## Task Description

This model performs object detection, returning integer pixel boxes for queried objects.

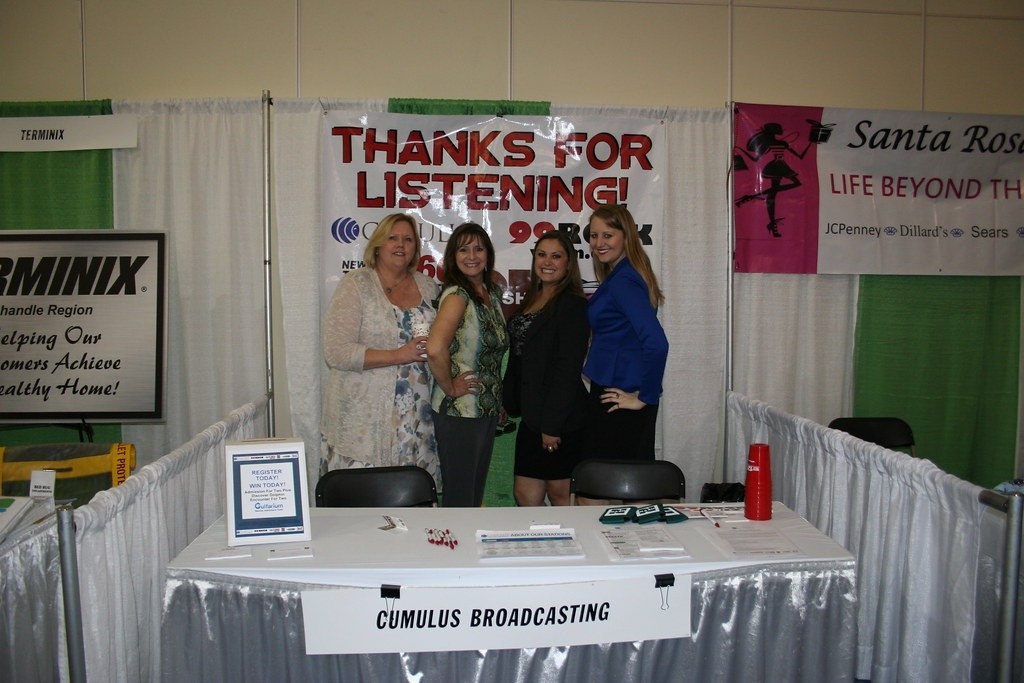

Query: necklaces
[377,272,408,293]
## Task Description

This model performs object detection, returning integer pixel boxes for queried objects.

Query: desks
[162,501,855,682]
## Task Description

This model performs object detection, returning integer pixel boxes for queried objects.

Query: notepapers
[637,540,684,552]
[529,520,561,530]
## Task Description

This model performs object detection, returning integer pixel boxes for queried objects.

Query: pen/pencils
[424,527,459,549]
[699,508,722,530]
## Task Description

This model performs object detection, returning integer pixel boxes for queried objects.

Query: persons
[424,224,510,508]
[319,212,444,508]
[575,204,669,506]
[502,231,591,506]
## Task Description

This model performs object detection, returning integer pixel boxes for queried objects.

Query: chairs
[829,417,916,459]
[315,465,439,507]
[569,459,686,501]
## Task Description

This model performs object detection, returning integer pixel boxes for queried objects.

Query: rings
[416,345,421,350]
[616,393,619,398]
[470,384,471,388]
[616,404,620,409]
[548,446,552,450]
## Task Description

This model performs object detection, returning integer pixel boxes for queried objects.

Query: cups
[745,443,773,521]
